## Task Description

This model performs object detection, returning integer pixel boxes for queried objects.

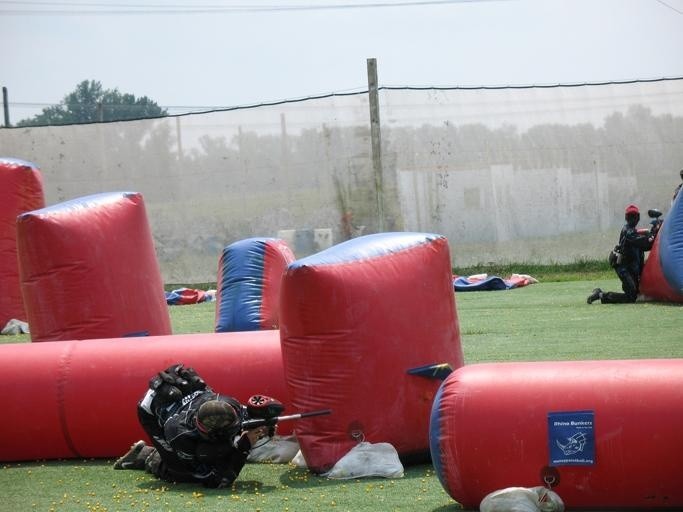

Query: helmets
[196,400,242,438]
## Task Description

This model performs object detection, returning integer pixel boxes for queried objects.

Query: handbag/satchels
[608,245,625,268]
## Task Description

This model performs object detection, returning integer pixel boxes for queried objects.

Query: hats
[625,204,639,215]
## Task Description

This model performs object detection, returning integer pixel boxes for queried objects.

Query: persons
[114,392,277,489]
[587,204,658,305]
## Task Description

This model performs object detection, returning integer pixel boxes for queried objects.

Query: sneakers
[114,440,145,469]
[587,288,602,304]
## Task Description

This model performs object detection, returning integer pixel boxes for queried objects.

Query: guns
[240,395,333,440]
[649,209,663,235]
